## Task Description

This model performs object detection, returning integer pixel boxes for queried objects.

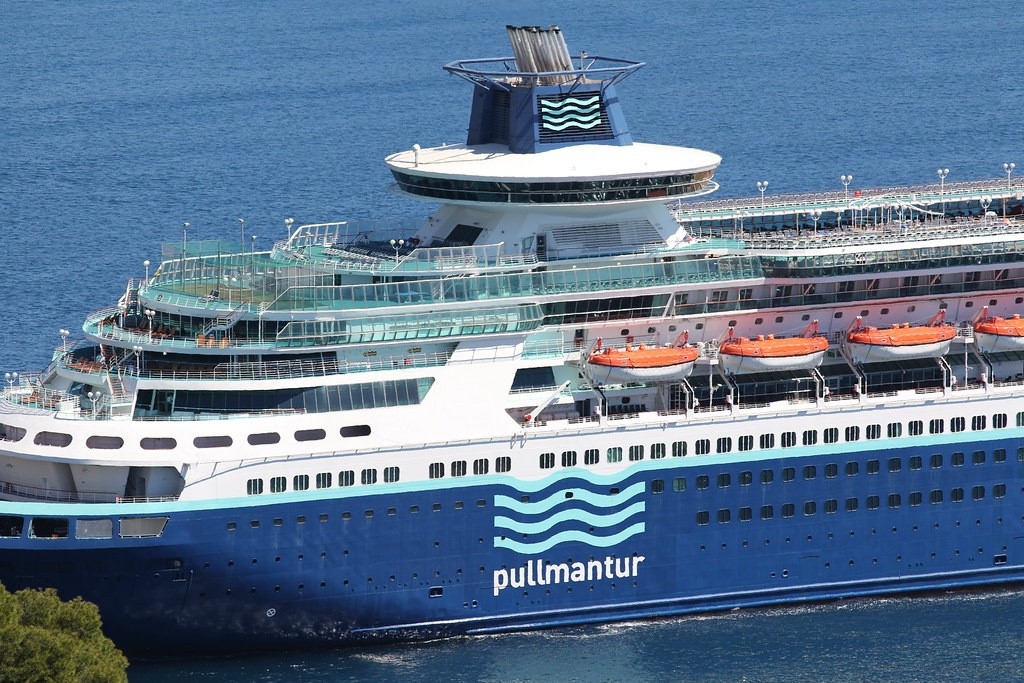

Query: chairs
[319,237,538,269]
[533,177,1024,301]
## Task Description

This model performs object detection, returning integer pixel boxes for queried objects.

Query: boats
[580,330,699,388]
[844,306,954,363]
[717,319,828,376]
[973,302,1024,351]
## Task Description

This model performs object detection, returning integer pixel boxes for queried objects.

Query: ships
[0,22,1022,637]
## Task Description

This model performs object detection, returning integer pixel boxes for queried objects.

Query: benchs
[65,356,102,374]
[127,318,176,342]
[18,391,63,409]
